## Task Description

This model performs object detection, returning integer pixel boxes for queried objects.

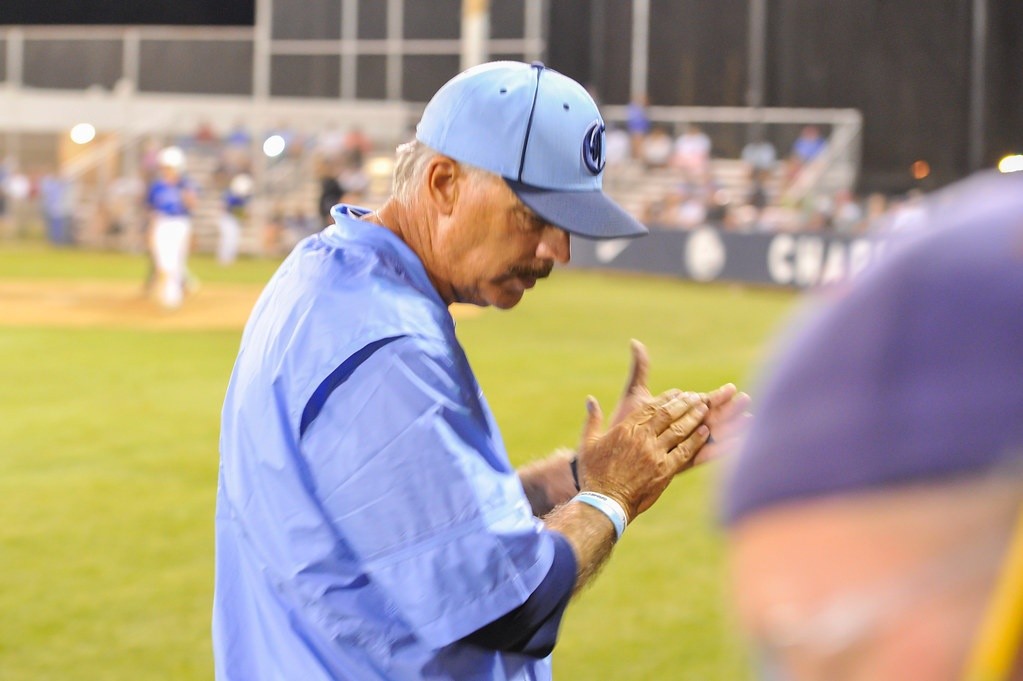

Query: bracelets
[571,457,581,492]
[571,488,628,541]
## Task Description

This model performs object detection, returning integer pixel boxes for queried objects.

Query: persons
[216,127,254,266]
[147,145,197,309]
[318,121,822,235]
[717,164,1022,681]
[40,165,79,242]
[213,58,755,681]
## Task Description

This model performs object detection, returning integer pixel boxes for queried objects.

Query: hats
[415,61,648,238]
